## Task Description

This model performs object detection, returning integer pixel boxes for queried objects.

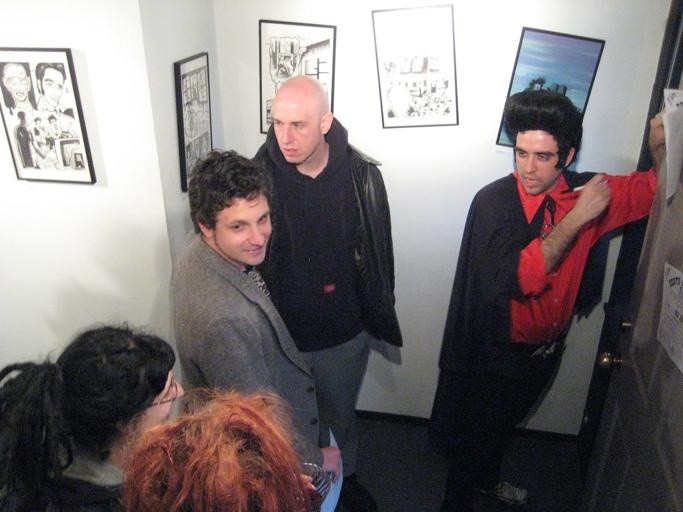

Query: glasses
[145,375,178,408]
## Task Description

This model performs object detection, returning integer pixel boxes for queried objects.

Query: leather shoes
[338,475,378,511]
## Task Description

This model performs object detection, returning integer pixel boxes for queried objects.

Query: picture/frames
[257,20,336,134]
[172,52,213,192]
[372,7,459,129]
[497,27,606,150]
[0,47,96,185]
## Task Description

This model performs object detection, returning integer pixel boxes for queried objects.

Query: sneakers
[492,481,528,507]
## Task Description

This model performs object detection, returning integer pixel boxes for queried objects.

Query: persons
[124,388,315,512]
[173,151,341,512]
[428,90,663,506]
[3,326,185,511]
[249,76,404,511]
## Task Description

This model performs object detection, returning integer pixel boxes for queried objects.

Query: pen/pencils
[573,179,608,193]
